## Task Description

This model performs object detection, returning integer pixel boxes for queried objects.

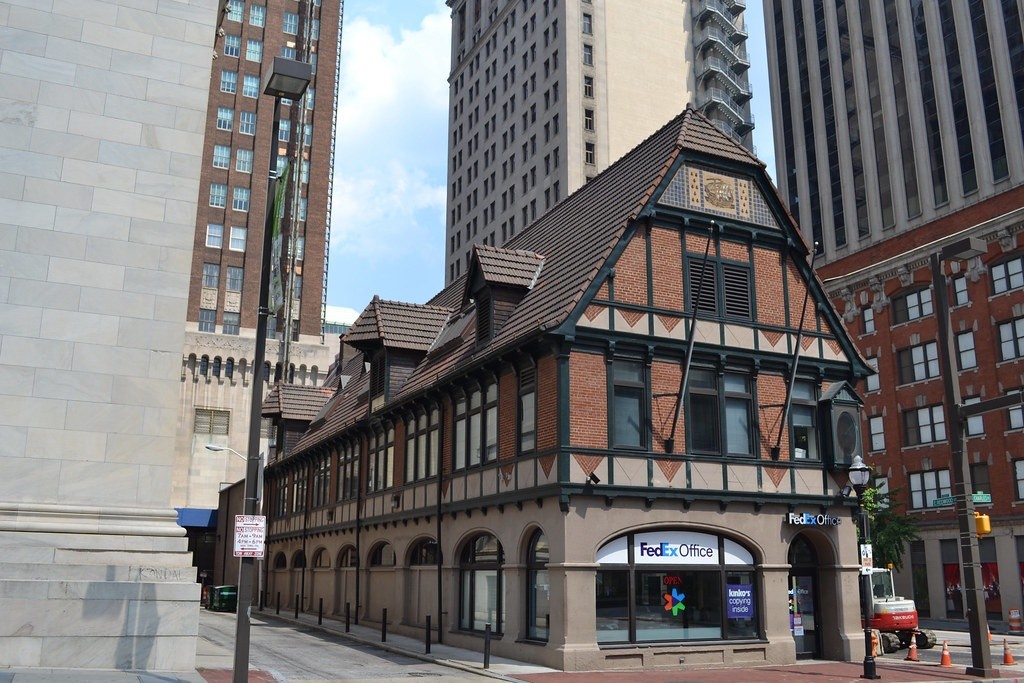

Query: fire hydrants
[871,631,878,658]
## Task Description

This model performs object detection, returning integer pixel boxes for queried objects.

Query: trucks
[206,585,237,613]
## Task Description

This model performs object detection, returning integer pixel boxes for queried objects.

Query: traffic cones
[936,640,953,668]
[987,623,994,643]
[903,634,921,662]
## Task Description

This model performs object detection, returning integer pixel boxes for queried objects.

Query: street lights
[205,56,312,683]
[928,248,1000,679]
[847,454,881,681]
[999,639,1017,666]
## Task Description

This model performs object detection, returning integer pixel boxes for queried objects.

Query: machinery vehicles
[858,568,938,655]
